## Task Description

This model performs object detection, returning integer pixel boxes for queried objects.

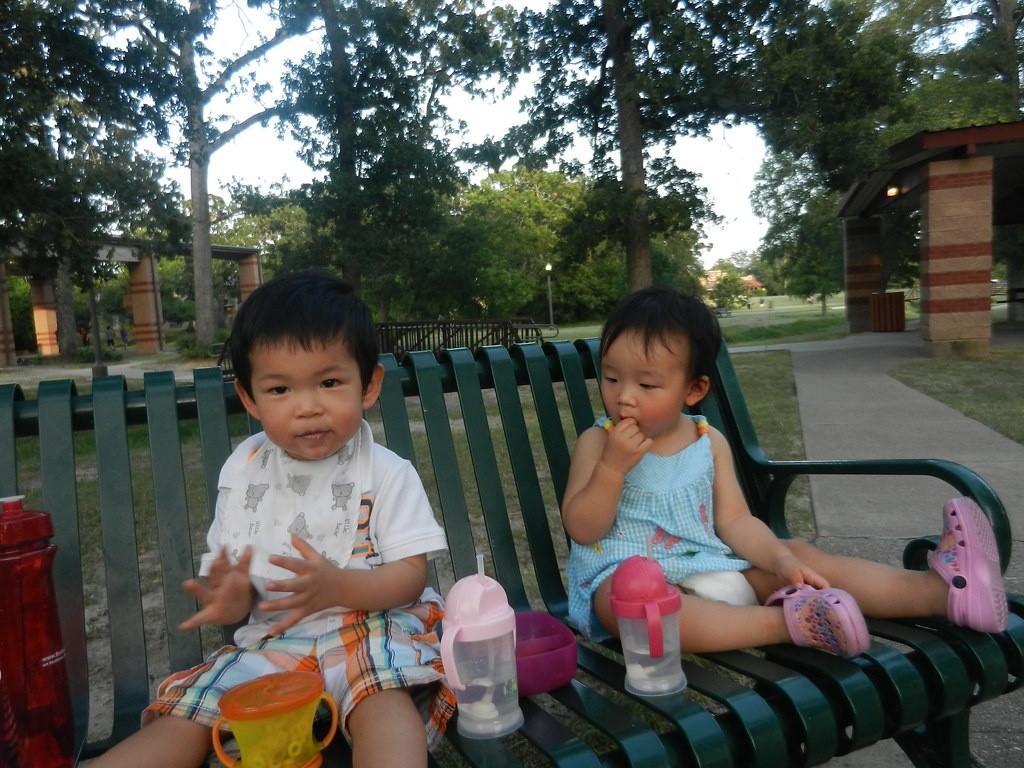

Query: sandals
[762,583,870,657]
[925,497,1009,635]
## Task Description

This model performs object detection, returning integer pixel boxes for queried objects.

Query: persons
[806,293,814,303]
[710,304,722,318]
[65,255,453,768]
[550,286,1014,662]
[759,296,765,307]
[79,326,91,348]
[104,323,116,350]
[120,323,128,350]
[746,295,752,310]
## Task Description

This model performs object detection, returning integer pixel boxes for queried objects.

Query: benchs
[0,337,1024,768]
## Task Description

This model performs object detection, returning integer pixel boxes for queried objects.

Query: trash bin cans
[871,290,906,333]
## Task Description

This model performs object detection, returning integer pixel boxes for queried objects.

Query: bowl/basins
[514,608,578,697]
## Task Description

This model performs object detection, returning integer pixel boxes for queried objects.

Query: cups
[610,536,694,695]
[436,554,524,740]
[212,670,338,768]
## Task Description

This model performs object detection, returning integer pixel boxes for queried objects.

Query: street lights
[545,262,556,330]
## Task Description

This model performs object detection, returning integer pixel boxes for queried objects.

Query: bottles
[0,491,79,767]
